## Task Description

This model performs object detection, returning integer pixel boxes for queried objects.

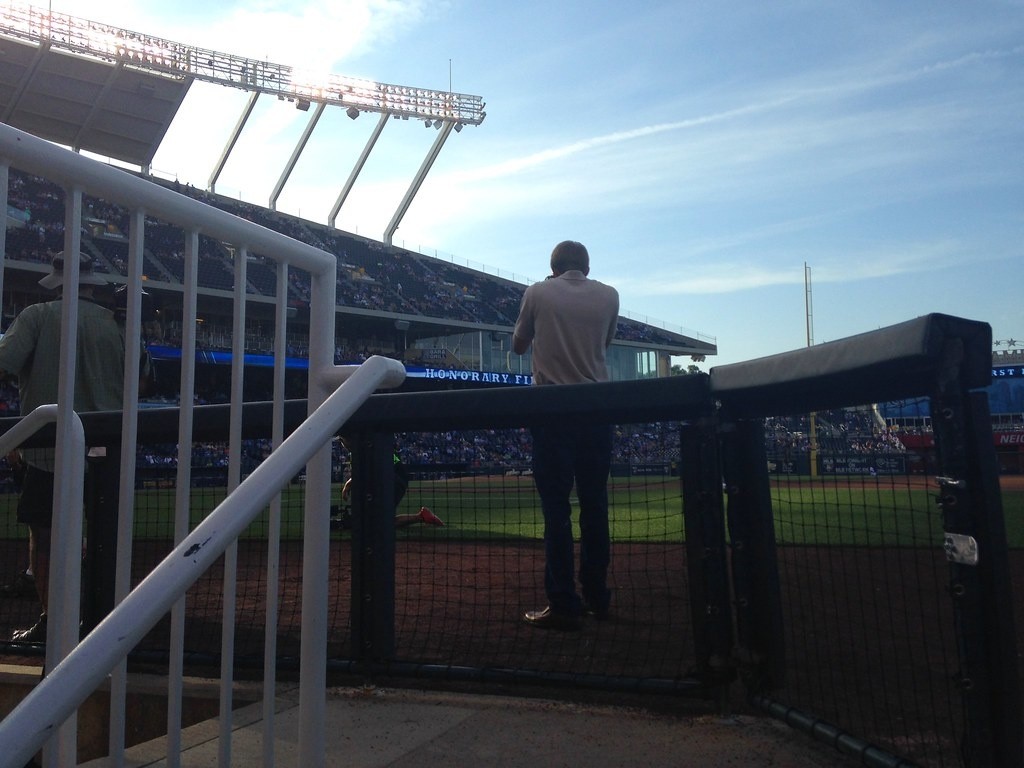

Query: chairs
[4,164,697,349]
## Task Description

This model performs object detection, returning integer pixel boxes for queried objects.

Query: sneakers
[420,507,445,526]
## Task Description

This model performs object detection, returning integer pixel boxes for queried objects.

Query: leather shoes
[523,604,579,631]
[583,607,609,620]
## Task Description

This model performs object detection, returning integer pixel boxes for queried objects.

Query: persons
[339,423,444,528]
[2,157,690,371]
[514,241,620,624]
[0,250,150,653]
[0,372,907,482]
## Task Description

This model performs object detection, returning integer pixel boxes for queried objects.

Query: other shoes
[11,620,50,645]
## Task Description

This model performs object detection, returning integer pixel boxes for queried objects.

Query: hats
[38,249,112,294]
